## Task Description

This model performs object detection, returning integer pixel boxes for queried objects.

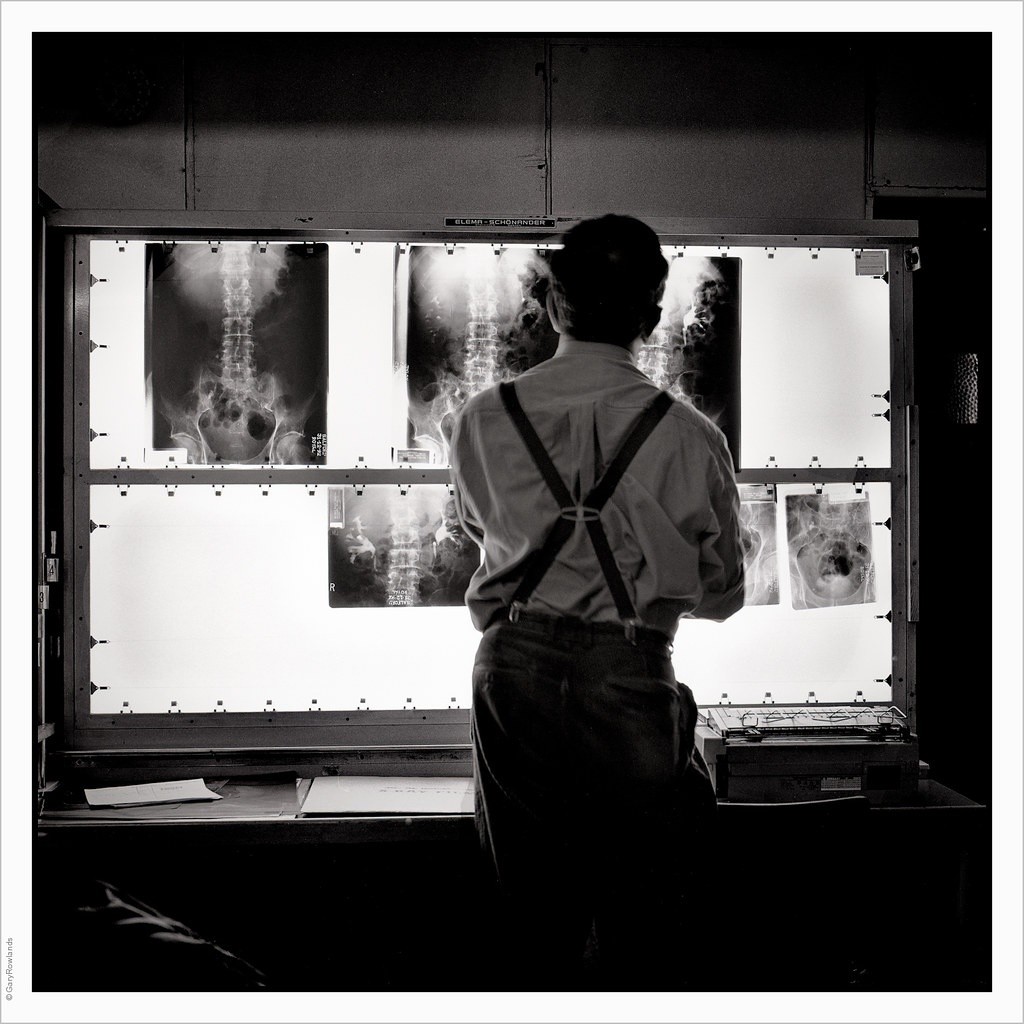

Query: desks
[37,771,998,841]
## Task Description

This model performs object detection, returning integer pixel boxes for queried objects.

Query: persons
[447,214,746,991]
